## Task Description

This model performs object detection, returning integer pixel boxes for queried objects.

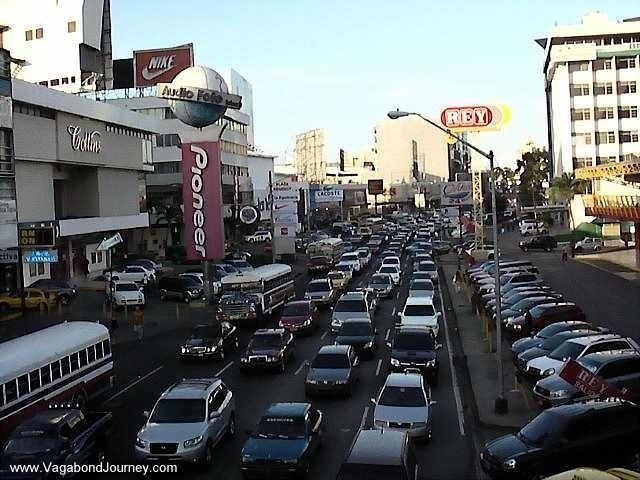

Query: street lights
[384,107,509,415]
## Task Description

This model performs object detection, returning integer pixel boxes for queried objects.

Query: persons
[524,306,532,336]
[561,244,568,264]
[453,270,462,294]
[132,305,145,341]
